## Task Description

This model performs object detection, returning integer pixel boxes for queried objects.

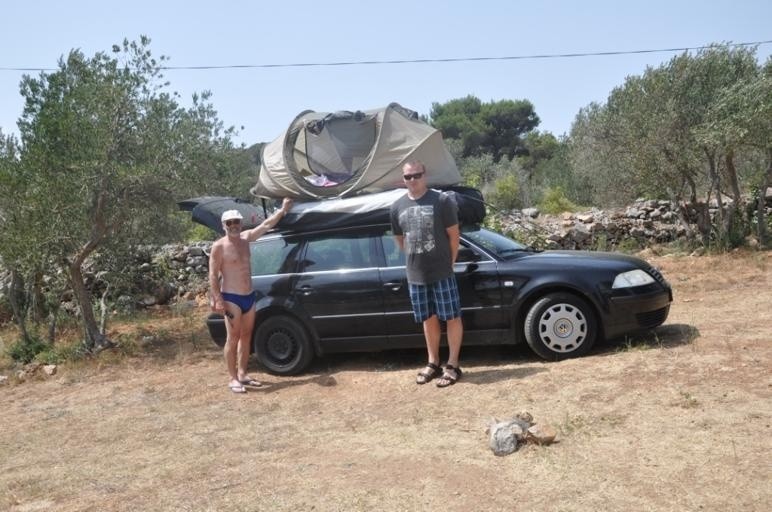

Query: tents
[248,101,466,200]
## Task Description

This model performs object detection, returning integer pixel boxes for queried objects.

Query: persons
[208,197,293,394]
[389,159,464,388]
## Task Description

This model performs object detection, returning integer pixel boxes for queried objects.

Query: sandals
[416,362,443,384]
[436,365,461,387]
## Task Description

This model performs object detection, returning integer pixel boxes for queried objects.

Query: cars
[175,194,673,376]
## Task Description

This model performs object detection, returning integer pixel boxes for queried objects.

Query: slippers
[239,378,262,386]
[229,382,247,393]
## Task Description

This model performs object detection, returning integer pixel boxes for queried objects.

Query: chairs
[381,238,403,266]
[304,249,346,271]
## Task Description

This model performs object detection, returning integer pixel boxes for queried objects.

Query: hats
[221,210,243,222]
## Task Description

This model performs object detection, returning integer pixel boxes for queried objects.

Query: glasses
[226,219,240,226]
[404,172,423,180]
